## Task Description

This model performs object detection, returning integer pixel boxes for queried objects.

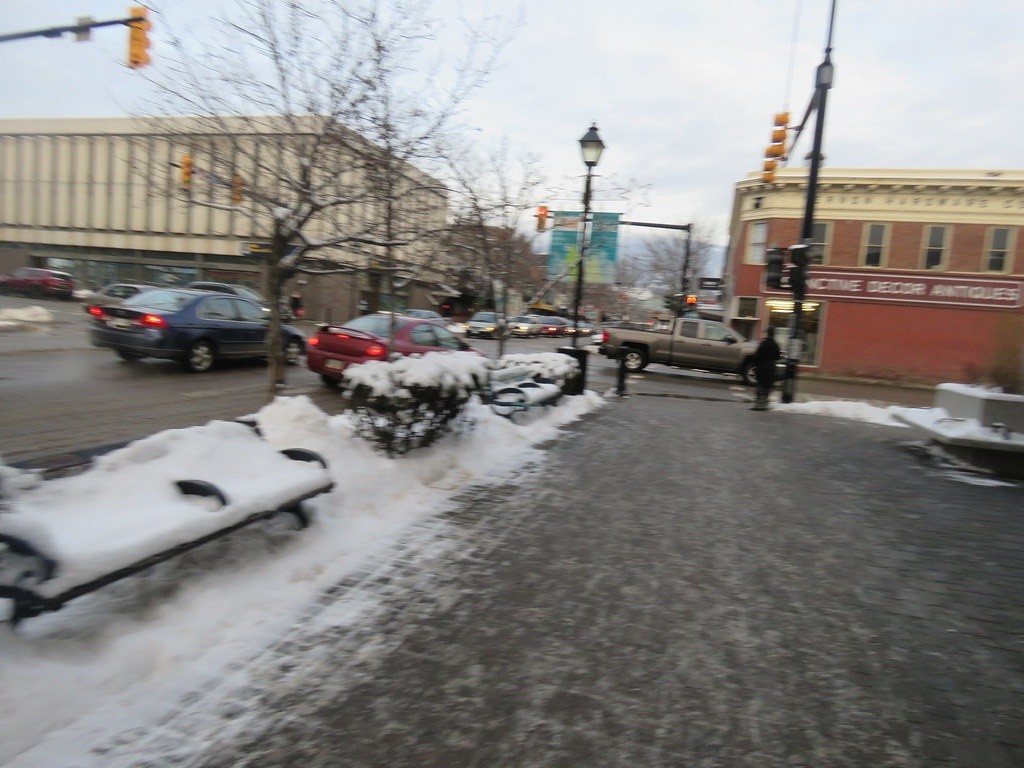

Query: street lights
[568,123,604,347]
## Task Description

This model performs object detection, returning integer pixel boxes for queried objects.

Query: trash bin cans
[556,346,589,395]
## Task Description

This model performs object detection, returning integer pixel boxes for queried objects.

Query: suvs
[185,280,292,325]
[0,267,74,299]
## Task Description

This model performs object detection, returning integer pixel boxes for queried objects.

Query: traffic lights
[762,147,776,184]
[181,156,193,192]
[664,293,671,309]
[232,174,244,203]
[770,110,791,155]
[537,204,549,232]
[129,7,151,69]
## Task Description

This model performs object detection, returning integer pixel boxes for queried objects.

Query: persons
[747,325,781,412]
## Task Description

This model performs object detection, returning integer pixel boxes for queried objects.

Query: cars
[402,303,597,342]
[88,288,306,375]
[299,313,487,394]
[85,283,158,317]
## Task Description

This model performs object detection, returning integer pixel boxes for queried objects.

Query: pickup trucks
[599,315,793,385]
